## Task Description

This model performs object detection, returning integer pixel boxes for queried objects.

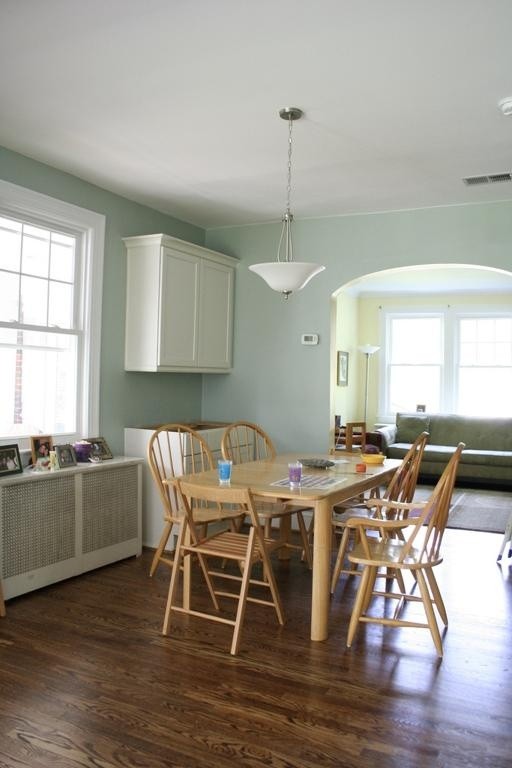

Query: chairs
[147,424,252,578]
[163,480,287,655]
[221,421,313,583]
[332,432,429,599]
[347,422,366,452]
[346,441,466,658]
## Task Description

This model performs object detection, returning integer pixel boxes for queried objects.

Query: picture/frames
[0,443,22,476]
[29,434,53,465]
[337,350,349,386]
[54,445,77,468]
[83,436,112,459]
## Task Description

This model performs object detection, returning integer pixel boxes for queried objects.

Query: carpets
[368,479,512,535]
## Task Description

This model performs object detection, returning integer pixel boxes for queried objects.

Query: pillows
[396,415,430,444]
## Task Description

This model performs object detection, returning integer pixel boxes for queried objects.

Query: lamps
[357,344,381,422]
[248,106,325,300]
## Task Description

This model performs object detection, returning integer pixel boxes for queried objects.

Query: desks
[334,434,364,444]
[335,444,366,452]
[161,452,408,642]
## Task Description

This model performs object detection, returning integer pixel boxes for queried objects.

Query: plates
[297,458,335,467]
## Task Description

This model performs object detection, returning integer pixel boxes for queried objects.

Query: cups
[288,463,302,487]
[216,460,233,485]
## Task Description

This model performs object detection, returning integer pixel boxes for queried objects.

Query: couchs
[375,413,512,487]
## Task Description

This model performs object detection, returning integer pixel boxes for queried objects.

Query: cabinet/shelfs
[122,234,237,373]
[124,423,255,554]
[0,454,143,600]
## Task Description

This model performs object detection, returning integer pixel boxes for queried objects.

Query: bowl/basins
[359,452,386,464]
[356,464,366,472]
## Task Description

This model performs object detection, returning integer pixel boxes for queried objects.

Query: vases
[72,443,92,463]
